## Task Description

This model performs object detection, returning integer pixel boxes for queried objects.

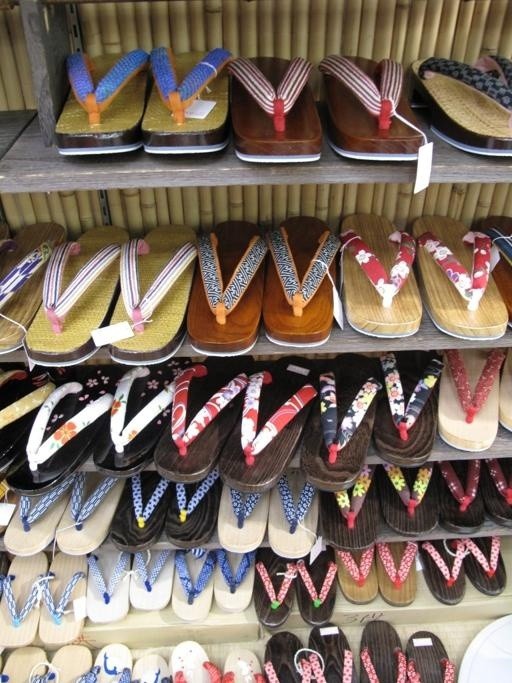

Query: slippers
[186,220,269,358]
[261,216,342,348]
[412,213,512,342]
[339,213,423,339]
[318,54,424,163]
[227,56,323,164]
[0,216,65,355]
[412,55,512,158]
[54,49,149,157]
[107,225,198,367]
[141,46,231,155]
[22,223,130,368]
